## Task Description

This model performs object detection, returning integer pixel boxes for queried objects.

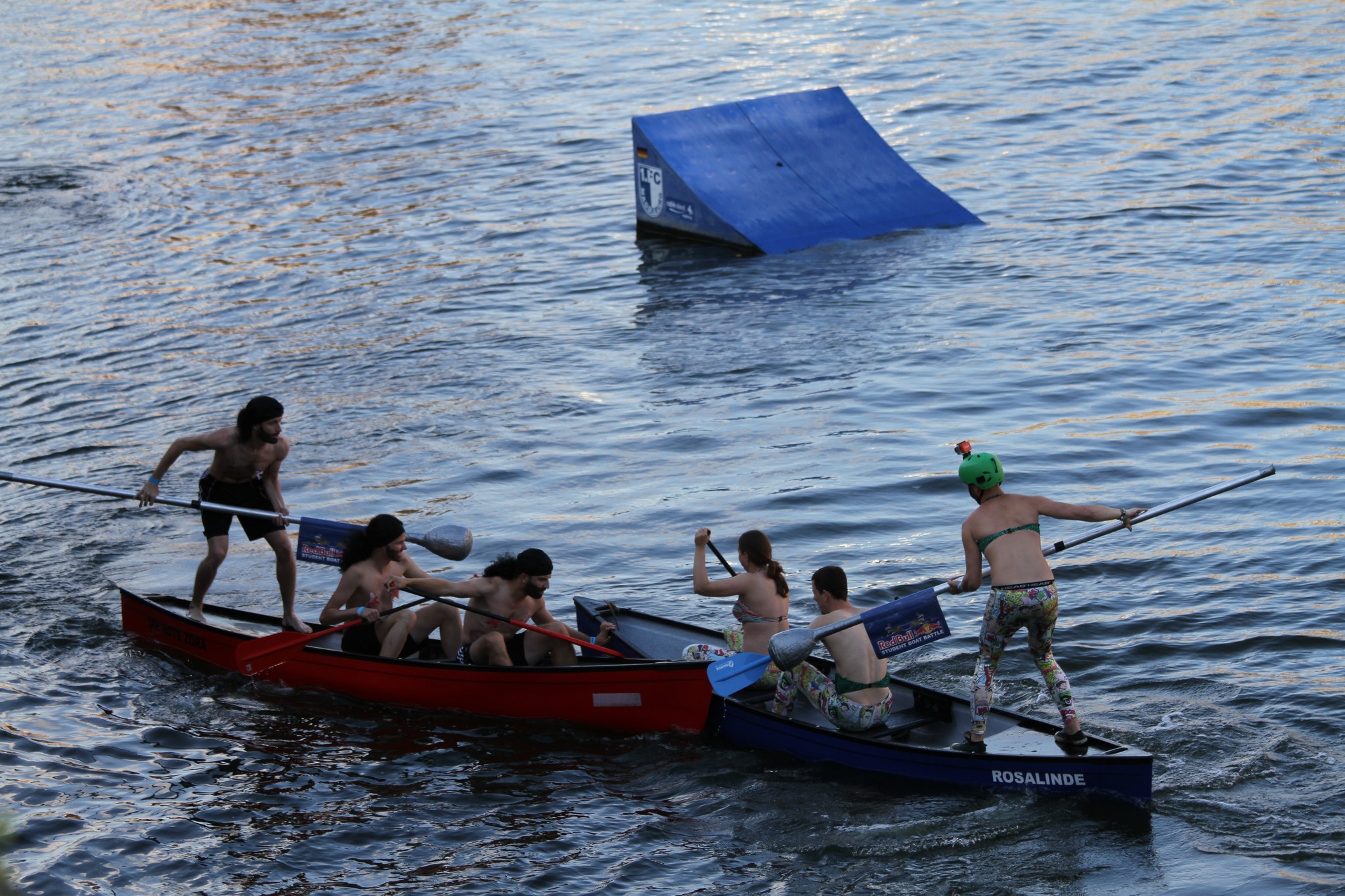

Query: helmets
[955,441,1004,489]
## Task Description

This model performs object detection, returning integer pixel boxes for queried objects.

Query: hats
[246,397,283,424]
[517,548,553,576]
[367,514,405,547]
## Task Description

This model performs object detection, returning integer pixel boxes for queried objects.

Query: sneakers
[1055,728,1087,745]
[950,731,987,753]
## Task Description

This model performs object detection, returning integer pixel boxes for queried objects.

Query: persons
[771,565,893,732]
[383,548,616,667]
[947,452,1148,754]
[319,514,481,658]
[682,527,790,689]
[132,396,313,634]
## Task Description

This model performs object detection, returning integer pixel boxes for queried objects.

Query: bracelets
[1117,508,1126,522]
[590,637,596,644]
[149,476,160,484]
[957,582,962,592]
[357,606,365,617]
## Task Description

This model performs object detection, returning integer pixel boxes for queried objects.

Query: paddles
[235,573,479,676]
[389,582,629,658]
[707,596,899,696]
[707,530,737,577]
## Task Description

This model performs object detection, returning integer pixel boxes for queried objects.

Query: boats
[571,594,1155,805]
[114,582,716,737]
